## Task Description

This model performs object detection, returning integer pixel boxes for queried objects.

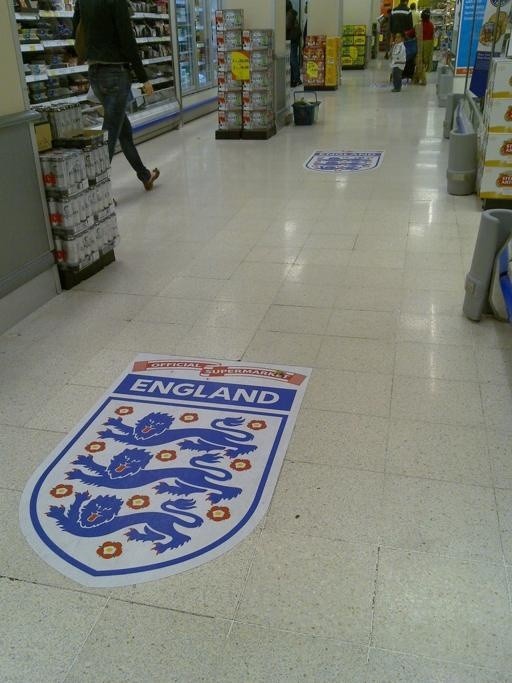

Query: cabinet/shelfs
[13,0,233,153]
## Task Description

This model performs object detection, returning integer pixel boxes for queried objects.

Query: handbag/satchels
[72,20,89,63]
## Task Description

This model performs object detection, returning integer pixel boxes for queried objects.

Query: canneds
[39,143,120,268]
[31,102,84,138]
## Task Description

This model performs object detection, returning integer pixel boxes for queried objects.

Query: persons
[70,0,160,210]
[376,0,437,93]
[285,0,304,88]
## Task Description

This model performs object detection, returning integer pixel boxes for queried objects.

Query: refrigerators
[170,0,223,124]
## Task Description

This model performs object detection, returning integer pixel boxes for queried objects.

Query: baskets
[291,89,323,126]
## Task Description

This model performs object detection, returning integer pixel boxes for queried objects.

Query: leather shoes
[142,167,160,192]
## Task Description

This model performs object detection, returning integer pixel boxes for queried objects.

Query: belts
[87,63,130,69]
